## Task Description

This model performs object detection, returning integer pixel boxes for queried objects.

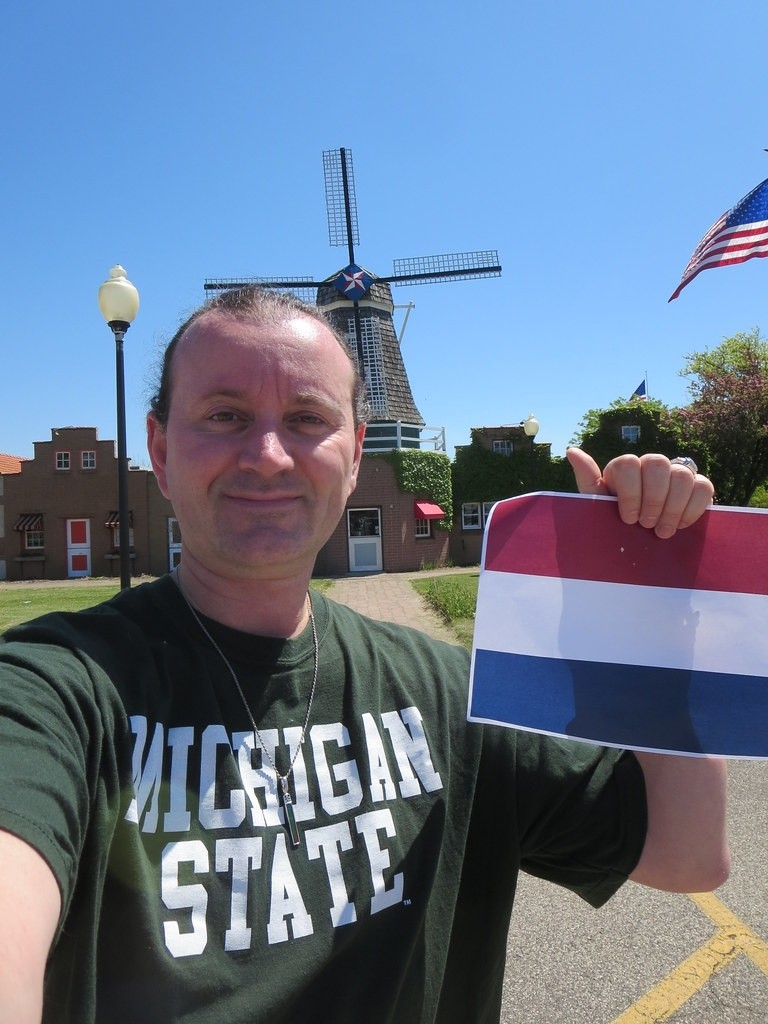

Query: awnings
[415,501,444,520]
[105,511,133,528]
[13,514,42,530]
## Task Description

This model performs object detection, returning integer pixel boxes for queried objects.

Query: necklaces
[175,568,318,845]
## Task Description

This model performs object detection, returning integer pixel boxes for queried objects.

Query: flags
[666,177,768,302]
[629,378,646,406]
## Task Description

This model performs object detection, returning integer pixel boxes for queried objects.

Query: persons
[0,288,728,1024]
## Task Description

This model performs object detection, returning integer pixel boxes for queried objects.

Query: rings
[671,456,697,477]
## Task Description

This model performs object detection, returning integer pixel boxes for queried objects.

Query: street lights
[525,412,539,492]
[96,264,142,593]
